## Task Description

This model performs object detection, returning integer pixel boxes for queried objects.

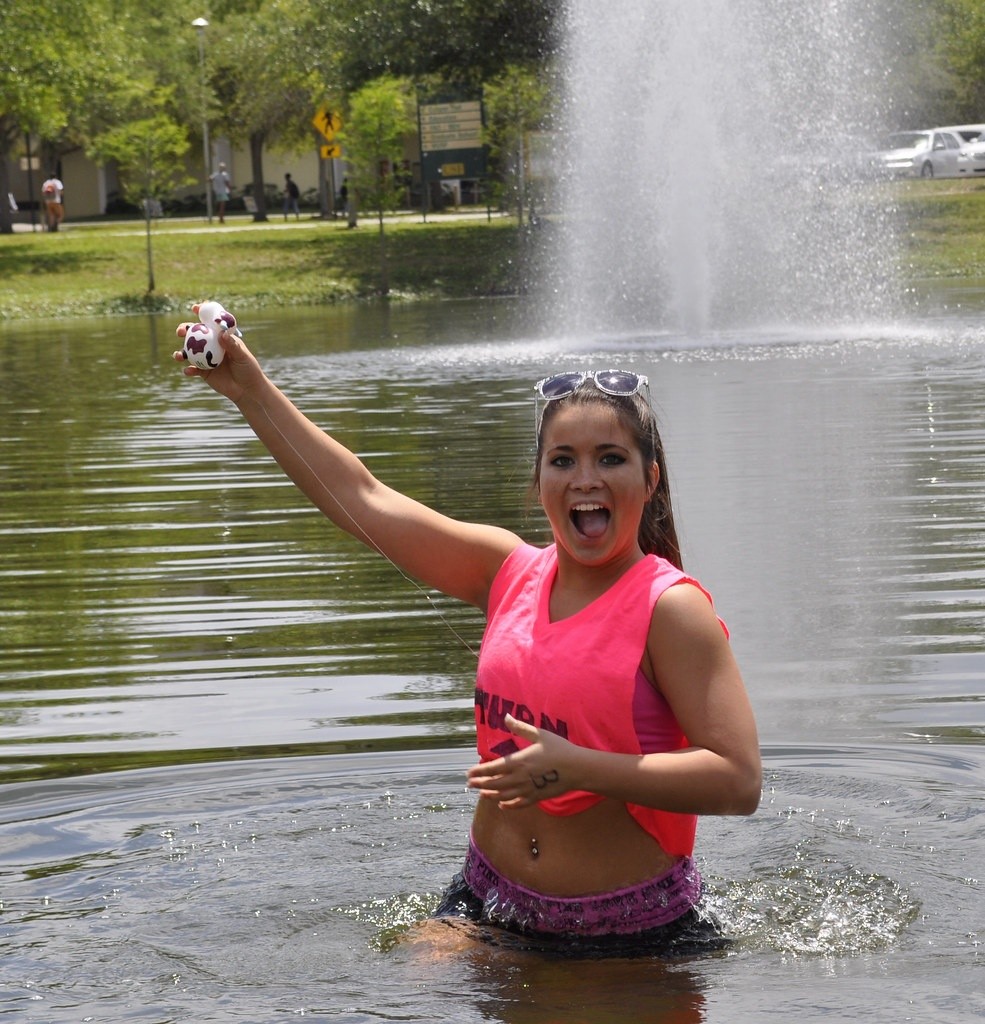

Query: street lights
[191,18,212,223]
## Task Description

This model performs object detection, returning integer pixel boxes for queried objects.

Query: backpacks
[44,180,57,199]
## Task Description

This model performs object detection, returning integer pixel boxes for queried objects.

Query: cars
[872,126,974,182]
[918,123,985,174]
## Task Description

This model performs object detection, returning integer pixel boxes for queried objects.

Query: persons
[41,172,64,231]
[283,174,300,221]
[174,304,762,933]
[340,178,349,218]
[207,162,235,224]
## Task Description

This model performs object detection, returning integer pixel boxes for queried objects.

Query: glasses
[534,369,657,462]
